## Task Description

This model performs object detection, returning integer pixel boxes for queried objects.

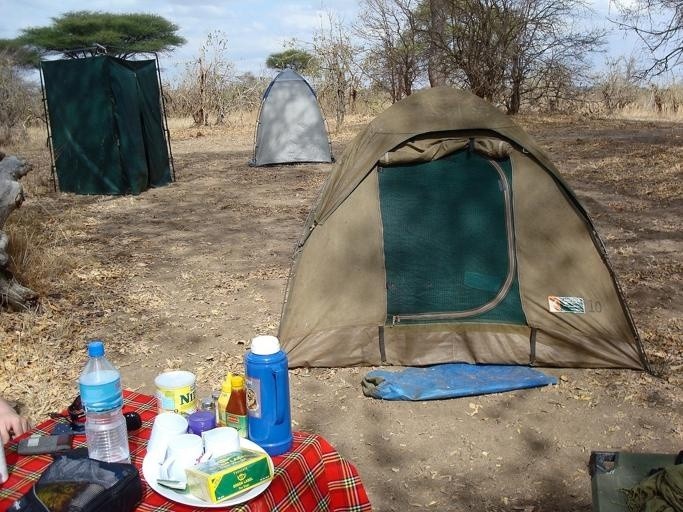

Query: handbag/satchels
[8,455,141,512]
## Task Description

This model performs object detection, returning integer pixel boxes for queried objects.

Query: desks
[0,390,376,512]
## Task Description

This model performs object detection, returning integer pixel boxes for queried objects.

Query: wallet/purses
[17,434,72,455]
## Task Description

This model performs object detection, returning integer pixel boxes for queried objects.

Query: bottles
[78,341,130,463]
[201,335,293,457]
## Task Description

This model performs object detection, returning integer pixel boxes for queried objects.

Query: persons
[0,400,31,443]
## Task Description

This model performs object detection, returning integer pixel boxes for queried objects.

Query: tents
[39,44,174,196]
[248,68,332,167]
[277,85,651,371]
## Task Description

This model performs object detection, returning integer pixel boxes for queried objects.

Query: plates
[142,438,275,508]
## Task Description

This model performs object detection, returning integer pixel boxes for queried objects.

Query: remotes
[52,411,142,435]
